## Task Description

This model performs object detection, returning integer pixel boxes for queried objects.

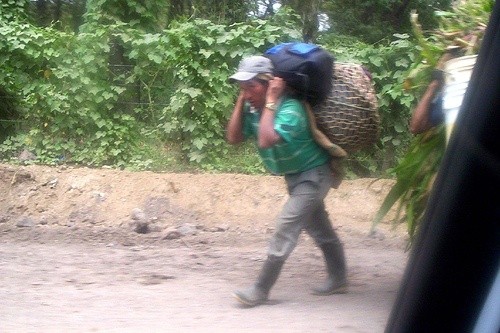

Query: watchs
[264,103,278,110]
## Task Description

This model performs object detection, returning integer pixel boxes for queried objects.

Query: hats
[228,56,273,84]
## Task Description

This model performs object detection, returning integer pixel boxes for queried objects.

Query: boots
[310,243,348,296]
[231,258,283,308]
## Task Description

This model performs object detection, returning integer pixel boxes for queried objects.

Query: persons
[409,52,451,136]
[225,55,350,309]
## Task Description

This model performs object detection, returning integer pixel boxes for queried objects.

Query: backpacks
[263,42,334,108]
